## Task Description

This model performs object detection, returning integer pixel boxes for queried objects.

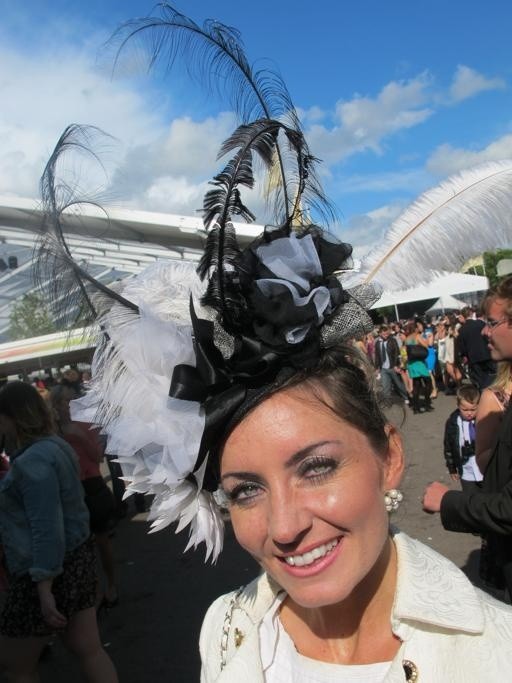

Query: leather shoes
[104,585,118,609]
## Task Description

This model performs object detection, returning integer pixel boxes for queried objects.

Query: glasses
[485,318,510,331]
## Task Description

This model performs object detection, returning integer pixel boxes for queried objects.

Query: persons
[444,385,482,489]
[2,366,140,679]
[422,274,509,602]
[351,306,495,412]
[199,348,511,682]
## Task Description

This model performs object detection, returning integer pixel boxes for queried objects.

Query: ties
[382,340,386,362]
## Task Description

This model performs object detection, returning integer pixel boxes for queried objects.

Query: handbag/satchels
[406,345,428,360]
